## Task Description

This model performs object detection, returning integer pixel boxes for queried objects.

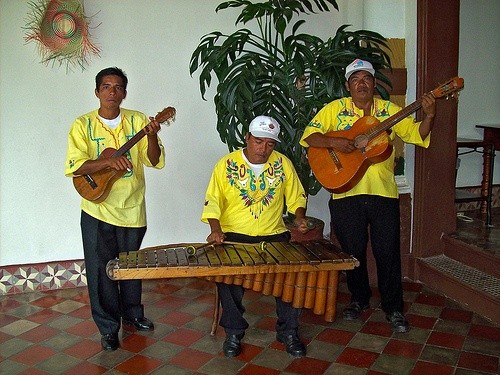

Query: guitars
[72,106,176,203]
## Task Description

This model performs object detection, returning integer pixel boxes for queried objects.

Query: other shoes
[385,311,408,333]
[341,299,371,321]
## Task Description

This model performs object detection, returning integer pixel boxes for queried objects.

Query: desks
[474,122,500,227]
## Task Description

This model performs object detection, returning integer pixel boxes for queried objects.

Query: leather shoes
[101,331,120,352]
[276,326,306,358]
[222,330,242,358]
[121,316,154,330]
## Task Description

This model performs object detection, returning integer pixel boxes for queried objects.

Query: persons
[200,116,308,358]
[299,58,435,333]
[65,67,165,351]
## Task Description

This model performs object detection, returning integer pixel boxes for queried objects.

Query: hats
[24,0,103,73]
[249,116,281,143]
[344,58,375,82]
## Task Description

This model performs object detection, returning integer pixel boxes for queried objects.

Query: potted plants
[188,0,394,241]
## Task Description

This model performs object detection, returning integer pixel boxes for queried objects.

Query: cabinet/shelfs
[457,135,494,227]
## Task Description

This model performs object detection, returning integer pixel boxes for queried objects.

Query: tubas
[305,77,465,195]
